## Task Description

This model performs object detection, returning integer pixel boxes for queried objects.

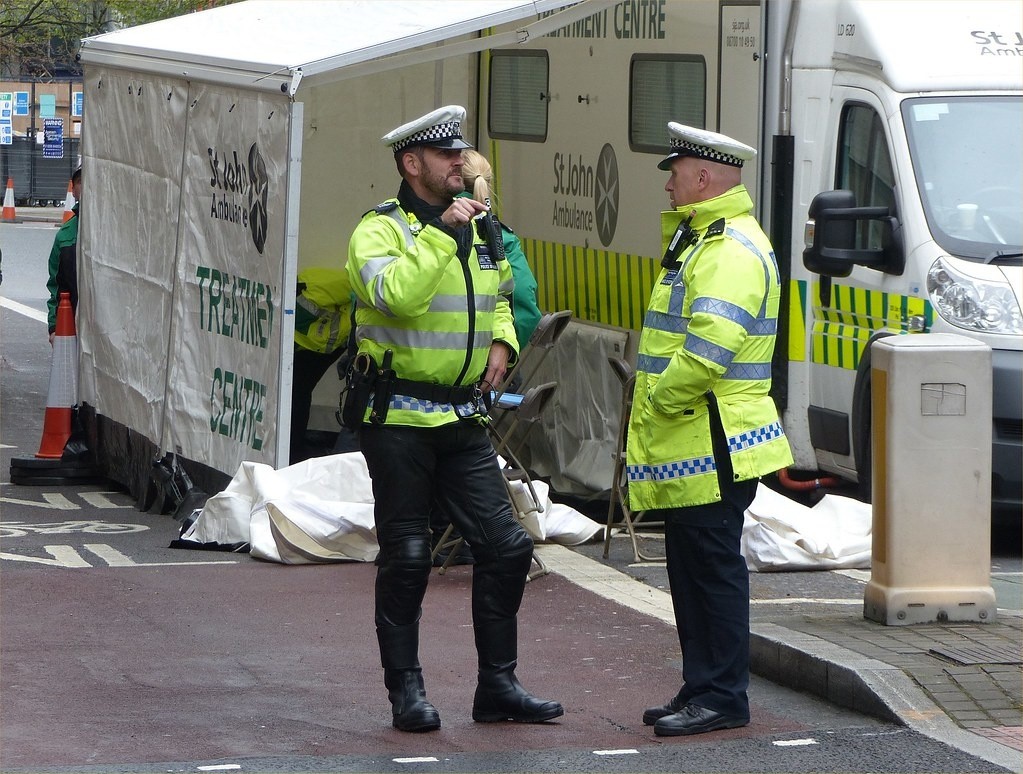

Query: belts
[392,375,477,406]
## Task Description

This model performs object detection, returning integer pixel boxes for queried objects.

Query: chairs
[427,307,575,583]
[602,355,665,563]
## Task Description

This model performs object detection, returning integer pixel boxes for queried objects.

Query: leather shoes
[643,690,691,725]
[383,666,441,731]
[471,662,564,724]
[654,703,751,737]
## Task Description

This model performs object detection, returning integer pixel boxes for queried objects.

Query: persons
[289,104,565,732]
[625,121,783,737]
[46,170,81,348]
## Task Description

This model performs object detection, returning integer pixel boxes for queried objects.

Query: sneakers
[429,526,475,567]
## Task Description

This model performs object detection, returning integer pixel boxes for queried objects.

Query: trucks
[477,1,1023,534]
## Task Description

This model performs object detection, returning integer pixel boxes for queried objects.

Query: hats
[380,104,475,150]
[656,121,758,171]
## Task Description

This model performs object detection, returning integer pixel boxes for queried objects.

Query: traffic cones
[0,177,23,223]
[35,293,78,457]
[54,180,75,227]
